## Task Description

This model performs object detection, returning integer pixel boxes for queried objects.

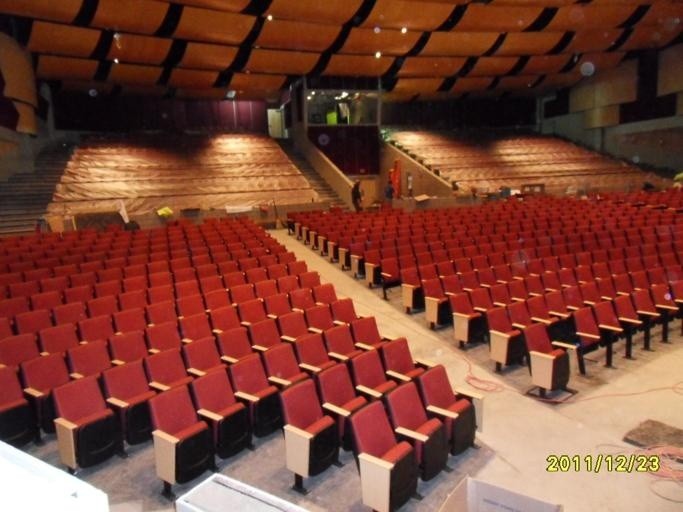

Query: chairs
[0,188,679,511]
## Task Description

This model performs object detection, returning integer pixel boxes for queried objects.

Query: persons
[406,171,413,197]
[383,180,394,206]
[452,180,458,191]
[351,180,363,214]
[641,181,656,193]
[471,185,478,199]
